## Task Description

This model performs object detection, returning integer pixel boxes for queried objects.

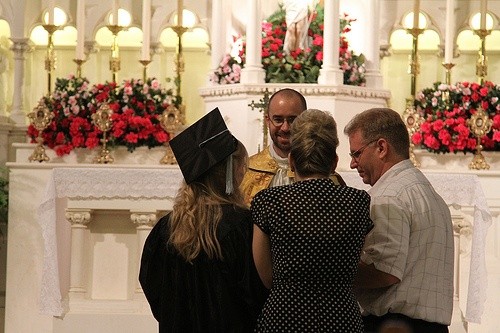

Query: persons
[250,108,374,333]
[344,107,454,333]
[139,107,270,333]
[239,89,347,210]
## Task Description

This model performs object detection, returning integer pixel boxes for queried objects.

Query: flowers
[209,5,367,86]
[26,73,186,161]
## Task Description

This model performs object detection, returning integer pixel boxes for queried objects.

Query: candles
[444,0,454,63]
[47,0,56,26]
[480,0,487,30]
[410,79,500,153]
[75,0,85,60]
[140,0,151,60]
[177,0,184,26]
[112,0,119,26]
[413,0,421,31]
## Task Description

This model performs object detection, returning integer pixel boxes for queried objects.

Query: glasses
[349,137,379,162]
[268,115,297,127]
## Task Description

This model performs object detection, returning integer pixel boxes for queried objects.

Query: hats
[169,107,239,195]
